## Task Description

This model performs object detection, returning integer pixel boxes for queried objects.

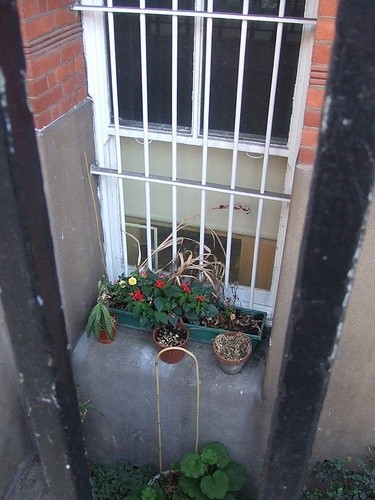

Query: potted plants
[142,436,245,500]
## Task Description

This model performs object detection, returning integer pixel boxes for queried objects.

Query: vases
[212,332,253,374]
[90,312,120,343]
[149,319,189,364]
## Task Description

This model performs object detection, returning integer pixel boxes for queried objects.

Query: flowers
[114,270,219,332]
